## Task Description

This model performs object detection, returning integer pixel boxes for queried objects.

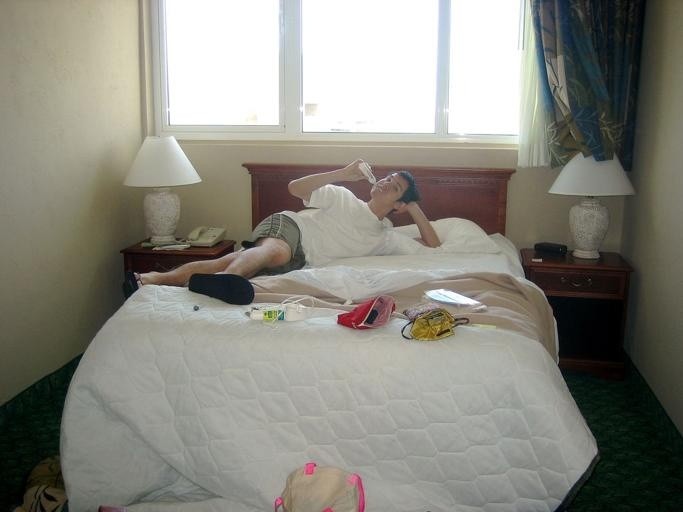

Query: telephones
[186,225,226,248]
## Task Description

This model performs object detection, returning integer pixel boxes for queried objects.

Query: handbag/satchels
[274,461,366,512]
[402,307,469,342]
[336,293,397,330]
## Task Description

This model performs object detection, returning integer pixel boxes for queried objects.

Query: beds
[58,161,602,512]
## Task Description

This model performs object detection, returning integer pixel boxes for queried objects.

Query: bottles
[358,163,378,186]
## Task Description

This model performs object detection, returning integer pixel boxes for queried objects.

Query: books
[422,288,487,314]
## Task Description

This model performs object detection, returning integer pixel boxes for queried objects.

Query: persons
[122,158,441,305]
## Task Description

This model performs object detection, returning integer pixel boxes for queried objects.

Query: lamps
[124,136,203,243]
[549,148,636,260]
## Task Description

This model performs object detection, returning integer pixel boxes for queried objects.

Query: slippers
[119,270,141,301]
[188,272,256,307]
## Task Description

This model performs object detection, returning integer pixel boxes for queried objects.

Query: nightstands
[120,238,236,290]
[521,248,635,373]
